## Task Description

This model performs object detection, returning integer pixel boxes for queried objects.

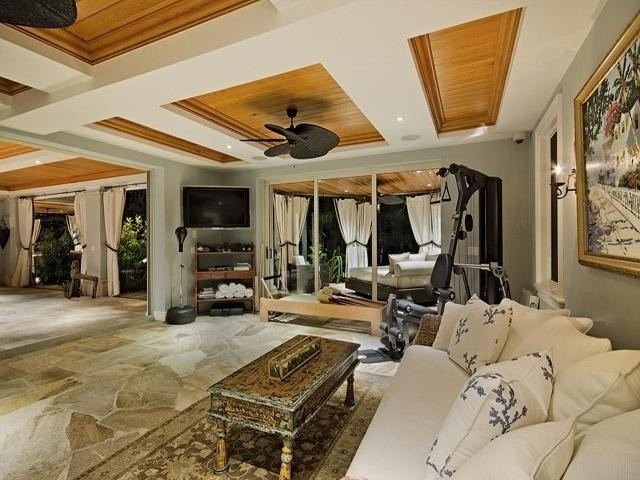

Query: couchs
[339,311,640,480]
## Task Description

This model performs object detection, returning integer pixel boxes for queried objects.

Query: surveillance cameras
[513,134,526,145]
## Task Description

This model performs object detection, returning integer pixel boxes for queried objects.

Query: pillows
[424,292,639,480]
[387,251,438,275]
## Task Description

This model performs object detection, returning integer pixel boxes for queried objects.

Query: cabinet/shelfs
[195,240,257,315]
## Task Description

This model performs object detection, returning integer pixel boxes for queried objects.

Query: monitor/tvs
[181,186,253,231]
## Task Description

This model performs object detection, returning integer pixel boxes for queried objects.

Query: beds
[345,261,438,303]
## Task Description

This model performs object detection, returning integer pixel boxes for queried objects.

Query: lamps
[239,107,341,159]
[548,162,576,199]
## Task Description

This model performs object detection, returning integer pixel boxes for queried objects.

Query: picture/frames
[574,10,640,279]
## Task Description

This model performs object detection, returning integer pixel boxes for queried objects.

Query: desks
[259,292,389,336]
